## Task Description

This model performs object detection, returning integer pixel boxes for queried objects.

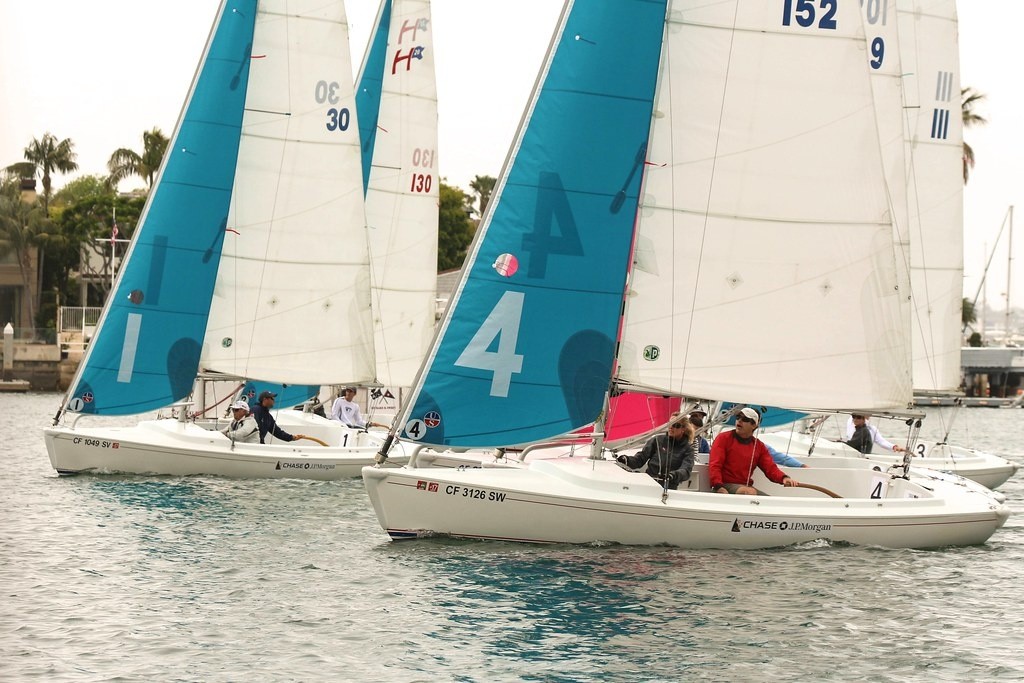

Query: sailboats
[43,0,440,480]
[360,0,1023,555]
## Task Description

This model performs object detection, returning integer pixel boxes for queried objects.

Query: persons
[220,401,260,445]
[250,392,305,444]
[836,415,904,454]
[708,407,812,496]
[619,413,694,490]
[686,403,710,454]
[332,386,379,431]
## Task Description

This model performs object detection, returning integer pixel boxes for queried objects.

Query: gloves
[617,455,629,463]
[659,473,673,482]
[366,422,372,428]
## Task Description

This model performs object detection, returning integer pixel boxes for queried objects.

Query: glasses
[672,421,685,429]
[851,414,862,419]
[232,409,239,413]
[271,397,275,400]
[346,390,352,393]
[735,414,755,424]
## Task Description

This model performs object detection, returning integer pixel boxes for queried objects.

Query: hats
[230,401,250,412]
[258,391,277,401]
[688,405,708,416]
[740,407,761,425]
[346,386,357,393]
